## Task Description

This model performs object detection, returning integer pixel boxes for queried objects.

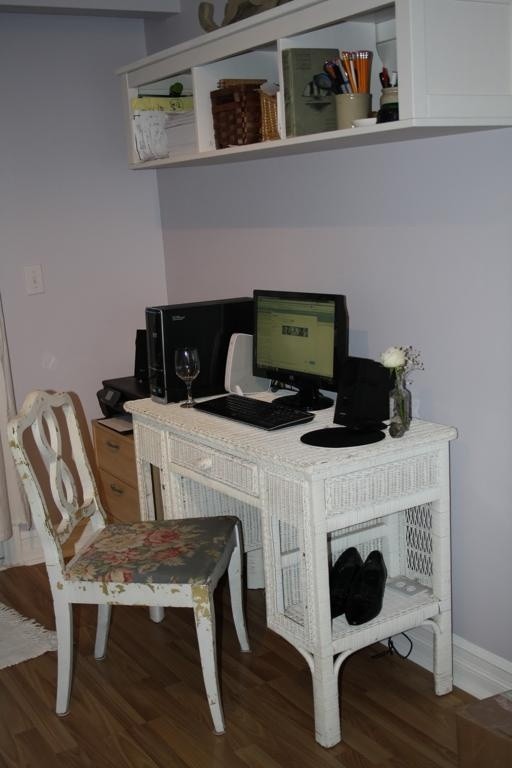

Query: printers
[96,376,145,434]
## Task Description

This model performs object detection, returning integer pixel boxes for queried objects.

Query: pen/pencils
[379,67,398,88]
[325,50,369,93]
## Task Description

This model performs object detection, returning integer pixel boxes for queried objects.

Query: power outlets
[26,267,44,295]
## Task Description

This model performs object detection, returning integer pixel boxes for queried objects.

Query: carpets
[0,602,76,670]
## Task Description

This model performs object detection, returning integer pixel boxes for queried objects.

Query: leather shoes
[346,551,386,625]
[330,547,363,618]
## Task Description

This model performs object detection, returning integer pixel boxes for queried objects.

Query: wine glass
[175,348,201,408]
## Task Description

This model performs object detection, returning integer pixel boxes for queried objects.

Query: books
[129,94,194,163]
[282,47,343,140]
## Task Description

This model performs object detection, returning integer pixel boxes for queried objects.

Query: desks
[122,394,457,750]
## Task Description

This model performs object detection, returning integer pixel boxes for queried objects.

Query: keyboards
[193,395,314,431]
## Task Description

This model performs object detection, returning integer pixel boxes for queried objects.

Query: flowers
[379,346,424,418]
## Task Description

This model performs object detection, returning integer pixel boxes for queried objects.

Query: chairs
[7,391,254,736]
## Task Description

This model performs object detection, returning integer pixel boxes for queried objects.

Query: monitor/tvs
[252,290,349,411]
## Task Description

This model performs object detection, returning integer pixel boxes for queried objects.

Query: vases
[388,379,411,432]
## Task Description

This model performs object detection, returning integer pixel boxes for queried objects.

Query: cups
[332,92,372,128]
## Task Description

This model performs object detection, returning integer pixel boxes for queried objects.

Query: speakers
[333,356,392,437]
[134,329,150,396]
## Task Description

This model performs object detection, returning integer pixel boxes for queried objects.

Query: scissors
[313,62,343,95]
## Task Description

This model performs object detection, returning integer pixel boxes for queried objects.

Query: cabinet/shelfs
[91,418,163,524]
[115,0,512,171]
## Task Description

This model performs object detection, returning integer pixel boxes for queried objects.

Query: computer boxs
[145,297,252,404]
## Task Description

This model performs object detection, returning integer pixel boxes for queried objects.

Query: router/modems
[224,333,271,394]
